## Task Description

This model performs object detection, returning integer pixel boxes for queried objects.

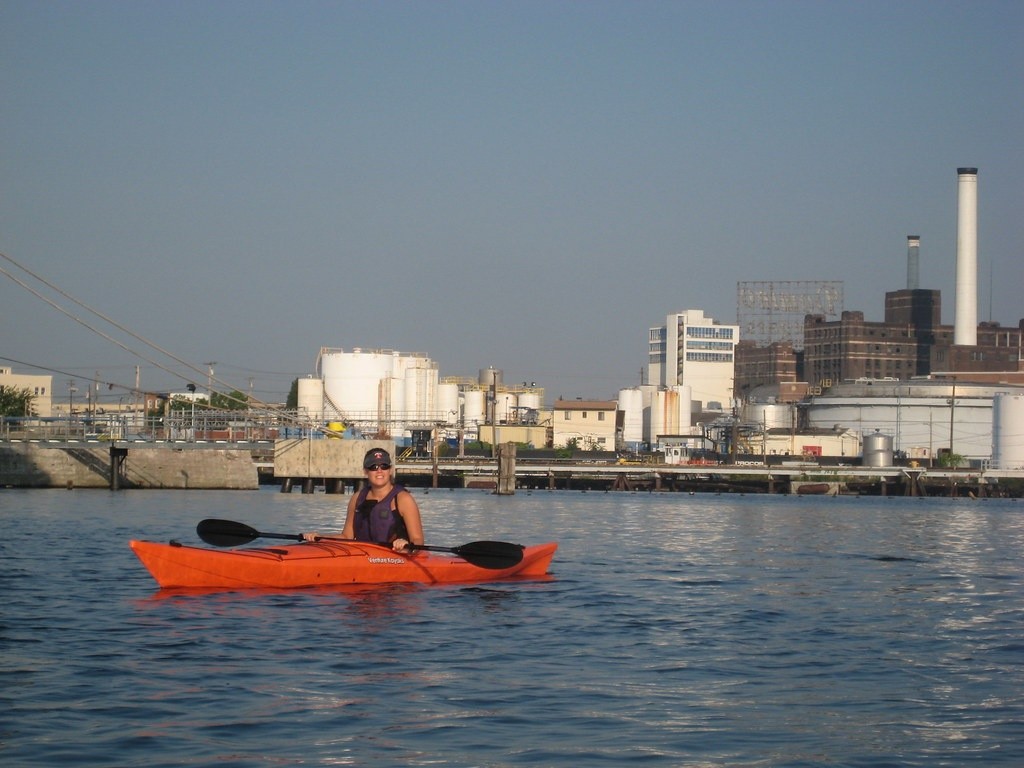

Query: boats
[129,537,558,590]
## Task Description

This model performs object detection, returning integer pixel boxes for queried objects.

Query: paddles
[196,517,524,570]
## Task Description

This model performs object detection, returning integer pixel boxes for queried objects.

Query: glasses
[366,465,392,471]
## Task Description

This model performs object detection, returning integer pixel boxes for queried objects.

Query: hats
[363,447,391,467]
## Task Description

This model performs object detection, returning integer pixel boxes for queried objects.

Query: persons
[302,448,426,556]
